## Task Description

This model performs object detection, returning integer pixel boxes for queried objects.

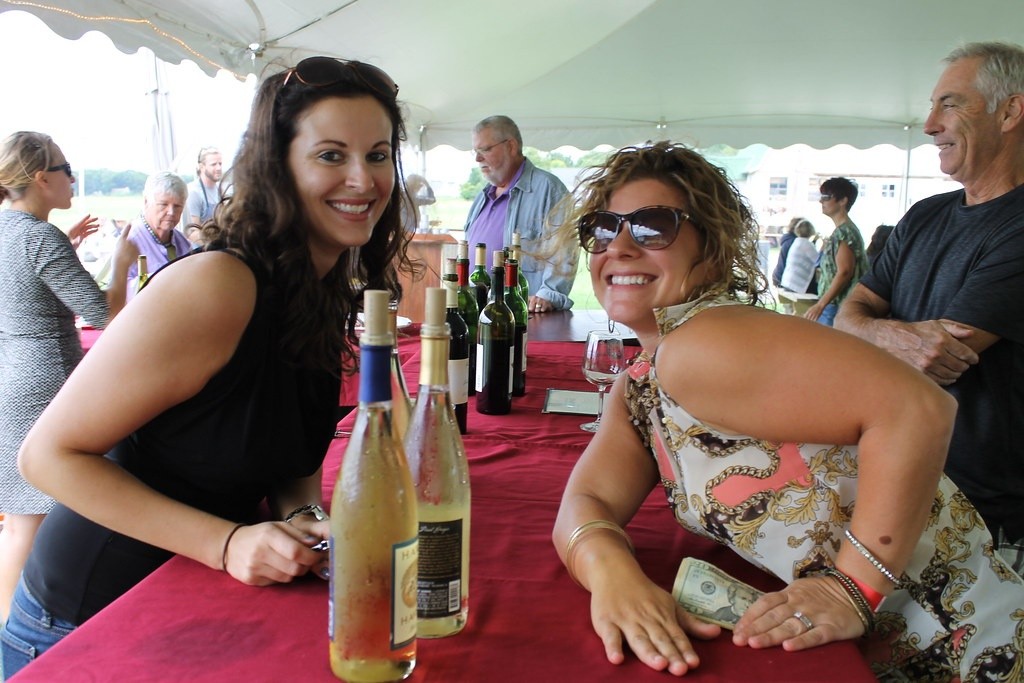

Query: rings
[793,612,813,631]
[536,304,541,307]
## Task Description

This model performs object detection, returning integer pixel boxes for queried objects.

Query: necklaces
[142,215,171,247]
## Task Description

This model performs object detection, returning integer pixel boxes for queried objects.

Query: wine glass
[579,330,625,432]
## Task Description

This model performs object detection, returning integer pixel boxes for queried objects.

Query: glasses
[472,137,511,157]
[281,56,400,97]
[577,205,704,253]
[821,194,837,201]
[43,162,72,176]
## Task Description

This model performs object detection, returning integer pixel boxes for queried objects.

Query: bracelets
[222,523,245,575]
[565,520,635,590]
[826,567,888,631]
[844,530,900,585]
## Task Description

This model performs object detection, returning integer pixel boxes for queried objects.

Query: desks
[2,328,881,683]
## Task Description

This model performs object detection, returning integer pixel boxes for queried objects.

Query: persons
[126,172,193,303]
[771,217,827,296]
[185,146,224,244]
[526,140,1024,683]
[0,57,419,683]
[0,131,139,622]
[400,174,436,233]
[463,115,581,312]
[831,42,1024,580]
[804,176,894,329]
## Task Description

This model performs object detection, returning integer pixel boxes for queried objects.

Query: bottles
[386,302,414,445]
[476,233,529,415]
[442,240,491,435]
[328,290,418,683]
[403,288,471,639]
[137,255,149,288]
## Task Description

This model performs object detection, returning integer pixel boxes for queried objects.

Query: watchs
[284,504,329,524]
